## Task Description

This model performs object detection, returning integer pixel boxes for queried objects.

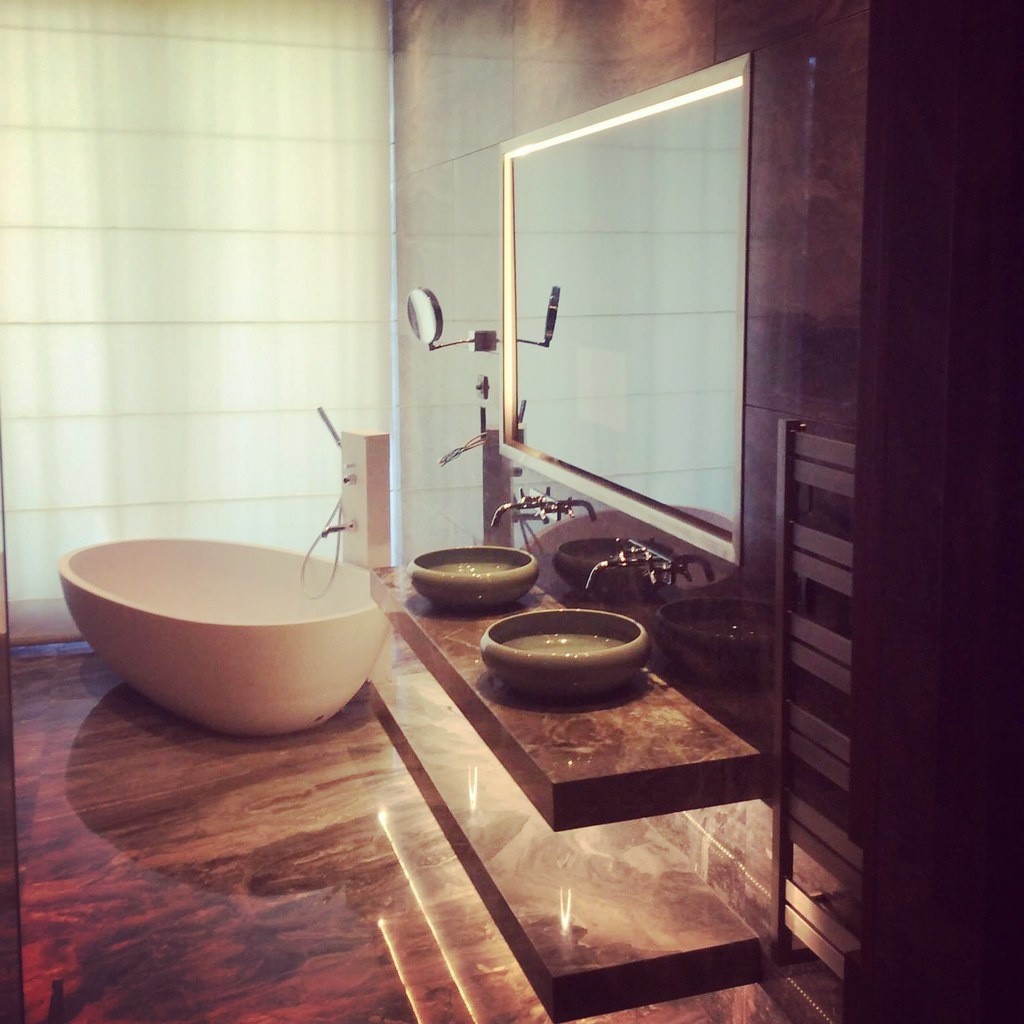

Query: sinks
[655,597,777,686]
[555,537,672,599]
[407,545,539,610]
[480,609,650,700]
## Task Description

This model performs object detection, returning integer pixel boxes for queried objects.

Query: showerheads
[318,404,342,447]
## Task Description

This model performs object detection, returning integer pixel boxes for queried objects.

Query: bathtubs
[57,537,391,738]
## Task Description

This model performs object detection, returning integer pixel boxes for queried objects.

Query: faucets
[638,535,715,582]
[490,487,561,527]
[542,486,596,522]
[318,525,346,536]
[584,538,675,590]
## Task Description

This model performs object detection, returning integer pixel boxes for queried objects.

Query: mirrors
[499,51,754,568]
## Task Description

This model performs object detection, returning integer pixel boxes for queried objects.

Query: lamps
[515,287,561,347]
[407,287,498,354]
